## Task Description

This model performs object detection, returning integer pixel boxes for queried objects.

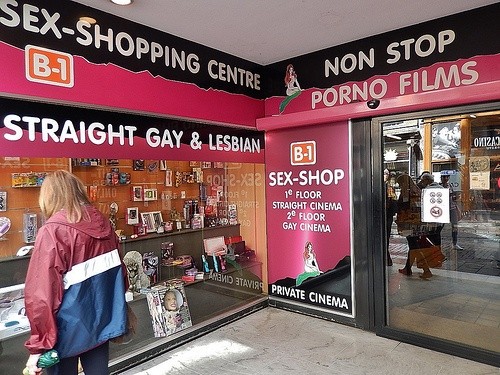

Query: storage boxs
[146,279,192,337]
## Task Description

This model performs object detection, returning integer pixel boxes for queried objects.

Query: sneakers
[452,243,463,249]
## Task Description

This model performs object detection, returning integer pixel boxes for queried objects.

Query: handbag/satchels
[450,204,461,223]
[109,304,136,345]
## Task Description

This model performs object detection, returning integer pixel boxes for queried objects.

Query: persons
[385,171,463,279]
[24,170,130,375]
[165,290,178,311]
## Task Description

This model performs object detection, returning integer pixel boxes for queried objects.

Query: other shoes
[419,270,432,278]
[399,266,412,275]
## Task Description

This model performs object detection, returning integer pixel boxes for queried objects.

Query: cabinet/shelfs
[0,222,262,375]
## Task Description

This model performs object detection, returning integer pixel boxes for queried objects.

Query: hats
[420,173,434,184]
[440,170,450,176]
